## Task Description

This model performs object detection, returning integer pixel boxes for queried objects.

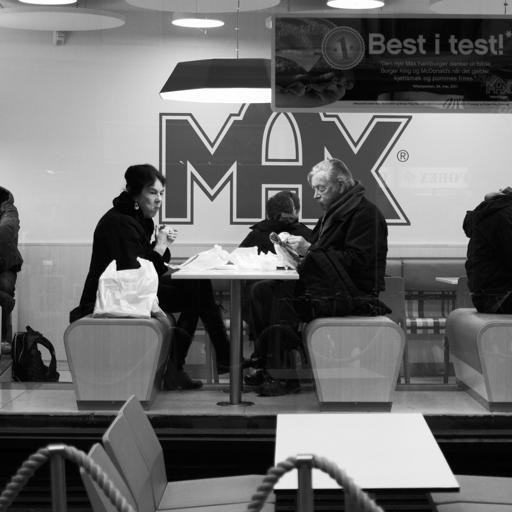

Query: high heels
[163,371,203,390]
[216,356,254,375]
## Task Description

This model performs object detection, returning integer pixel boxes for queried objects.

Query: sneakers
[252,377,301,396]
[243,369,272,386]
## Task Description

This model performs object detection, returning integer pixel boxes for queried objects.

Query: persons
[70,163,257,392]
[1,185,25,354]
[461,187,511,316]
[244,158,389,397]
[239,190,312,368]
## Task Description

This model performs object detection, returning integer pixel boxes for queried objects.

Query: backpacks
[293,245,377,322]
[10,326,59,383]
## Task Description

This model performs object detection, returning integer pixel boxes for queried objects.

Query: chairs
[434,503,512,511]
[102,414,276,512]
[81,443,138,511]
[121,398,276,509]
[427,474,512,504]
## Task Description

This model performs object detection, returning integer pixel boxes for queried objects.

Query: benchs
[304,314,407,411]
[62,313,174,404]
[441,307,511,408]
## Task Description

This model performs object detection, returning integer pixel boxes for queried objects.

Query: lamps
[172,0,225,29]
[325,0,385,12]
[159,1,272,105]
